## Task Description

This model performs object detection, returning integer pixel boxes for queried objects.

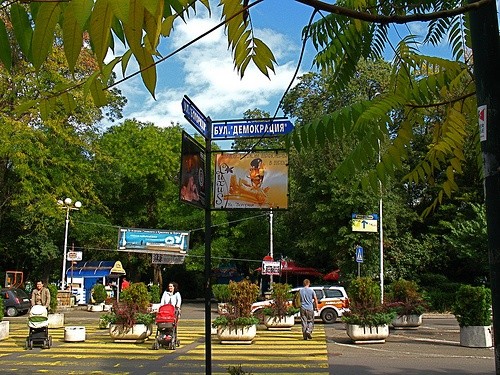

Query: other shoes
[306,332,312,339]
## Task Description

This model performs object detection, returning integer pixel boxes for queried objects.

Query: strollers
[25,304,53,350]
[154,307,180,350]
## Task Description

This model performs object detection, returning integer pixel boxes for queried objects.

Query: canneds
[266,266,279,273]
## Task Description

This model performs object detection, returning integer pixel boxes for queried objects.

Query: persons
[161,280,181,318]
[31,279,50,311]
[121,277,131,292]
[300,279,319,341]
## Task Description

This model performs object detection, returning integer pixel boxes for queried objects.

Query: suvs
[0,287,32,316]
[251,285,350,323]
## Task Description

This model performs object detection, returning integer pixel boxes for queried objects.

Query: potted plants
[88,283,160,343]
[211,280,299,344]
[0,286,10,340]
[382,277,428,329]
[450,284,493,348]
[46,284,64,328]
[341,277,396,343]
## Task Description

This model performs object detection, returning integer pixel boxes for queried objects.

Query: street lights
[55,198,83,289]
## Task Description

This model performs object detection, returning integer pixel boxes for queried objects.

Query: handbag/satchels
[292,289,302,309]
